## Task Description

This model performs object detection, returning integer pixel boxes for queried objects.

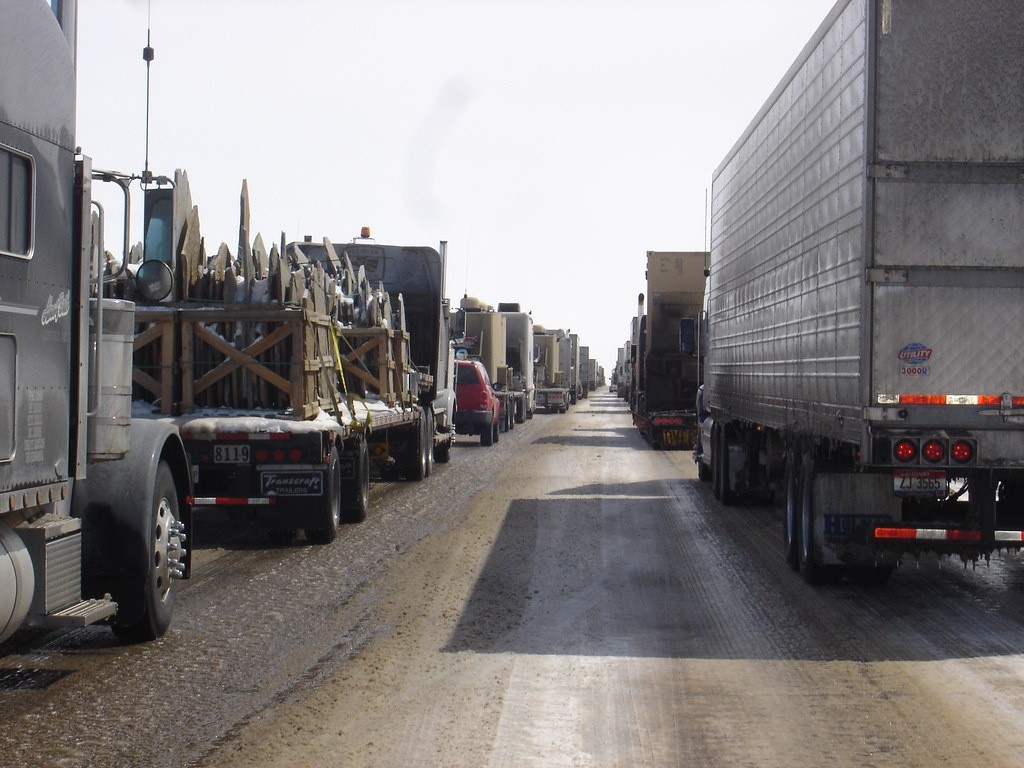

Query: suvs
[451,358,501,446]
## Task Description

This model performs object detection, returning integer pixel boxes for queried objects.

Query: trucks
[448,294,605,433]
[609,291,704,450]
[133,225,468,545]
[0,2,194,665]
[687,0,1023,581]
